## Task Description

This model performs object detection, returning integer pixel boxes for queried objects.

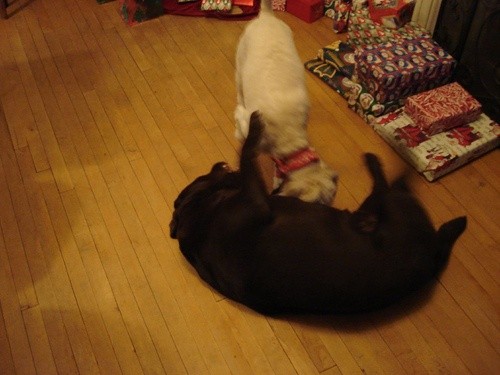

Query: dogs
[232,0,340,206]
[168,109,468,318]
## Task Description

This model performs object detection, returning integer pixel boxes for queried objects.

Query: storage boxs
[403,81,482,135]
[371,106,500,182]
[354,38,459,105]
[368,0,416,30]
[285,0,325,24]
